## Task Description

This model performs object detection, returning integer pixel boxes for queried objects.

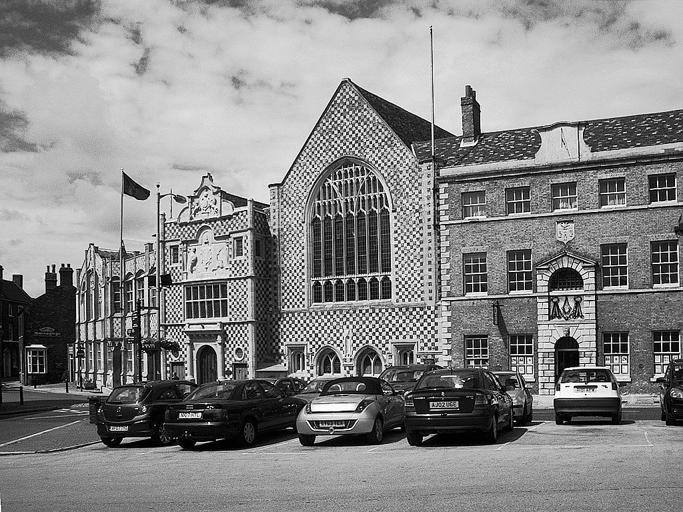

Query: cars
[402,364,513,445]
[553,366,627,425]
[376,364,446,407]
[488,371,533,425]
[249,377,308,396]
[96,380,199,447]
[288,377,349,403]
[295,377,405,446]
[162,378,307,449]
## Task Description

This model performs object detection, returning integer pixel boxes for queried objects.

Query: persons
[171,371,179,380]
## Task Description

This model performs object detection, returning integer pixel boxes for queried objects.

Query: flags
[121,173,151,201]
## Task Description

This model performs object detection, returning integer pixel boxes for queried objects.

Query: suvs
[655,359,683,426]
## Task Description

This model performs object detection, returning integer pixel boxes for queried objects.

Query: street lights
[155,182,187,380]
